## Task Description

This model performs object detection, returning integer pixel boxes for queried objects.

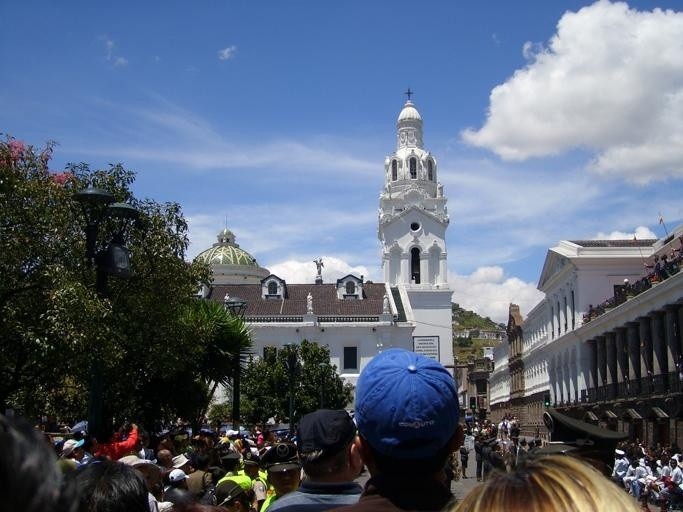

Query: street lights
[221,294,253,436]
[281,338,304,437]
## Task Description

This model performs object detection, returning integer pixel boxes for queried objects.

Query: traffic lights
[469,396,476,410]
[543,392,551,408]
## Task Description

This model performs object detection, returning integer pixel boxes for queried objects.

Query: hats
[512,438,543,445]
[547,406,630,457]
[242,451,260,467]
[479,435,498,448]
[117,455,153,466]
[63,438,85,457]
[355,348,460,445]
[226,430,261,437]
[615,448,626,456]
[221,454,241,461]
[213,481,245,507]
[297,408,356,453]
[168,454,190,481]
[261,440,299,472]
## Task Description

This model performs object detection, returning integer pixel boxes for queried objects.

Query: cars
[272,428,290,438]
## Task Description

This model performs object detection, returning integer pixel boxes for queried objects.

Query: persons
[2,406,683,512]
[326,347,464,512]
[581,236,682,327]
